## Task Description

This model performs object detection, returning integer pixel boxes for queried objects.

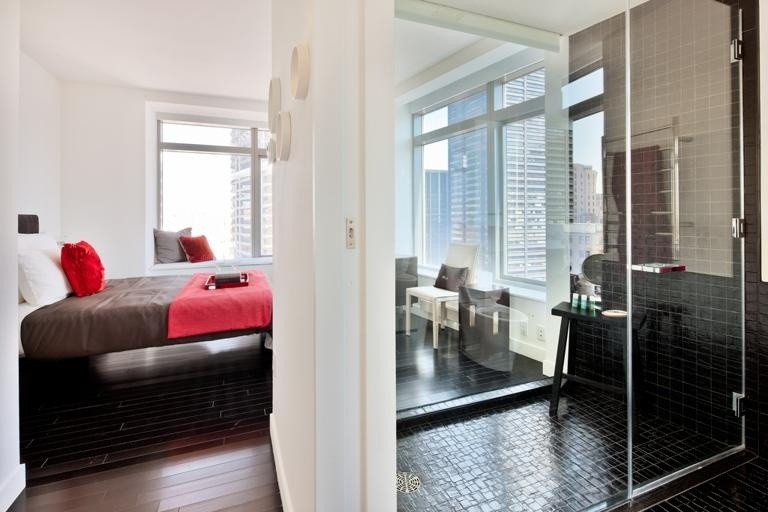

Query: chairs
[405,242,480,350]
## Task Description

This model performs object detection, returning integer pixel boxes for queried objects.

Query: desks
[549,302,647,430]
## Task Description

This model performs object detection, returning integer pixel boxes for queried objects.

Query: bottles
[571,292,596,312]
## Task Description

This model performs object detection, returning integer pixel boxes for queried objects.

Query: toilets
[461,252,528,372]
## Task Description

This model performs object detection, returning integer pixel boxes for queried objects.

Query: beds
[17,213,273,359]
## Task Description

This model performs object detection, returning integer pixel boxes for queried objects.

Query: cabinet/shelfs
[457,282,510,362]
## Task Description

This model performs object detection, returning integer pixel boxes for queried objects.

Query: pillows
[433,263,468,293]
[153,227,216,263]
[18,232,105,310]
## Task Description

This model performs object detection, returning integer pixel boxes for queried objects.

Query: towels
[611,144,674,280]
